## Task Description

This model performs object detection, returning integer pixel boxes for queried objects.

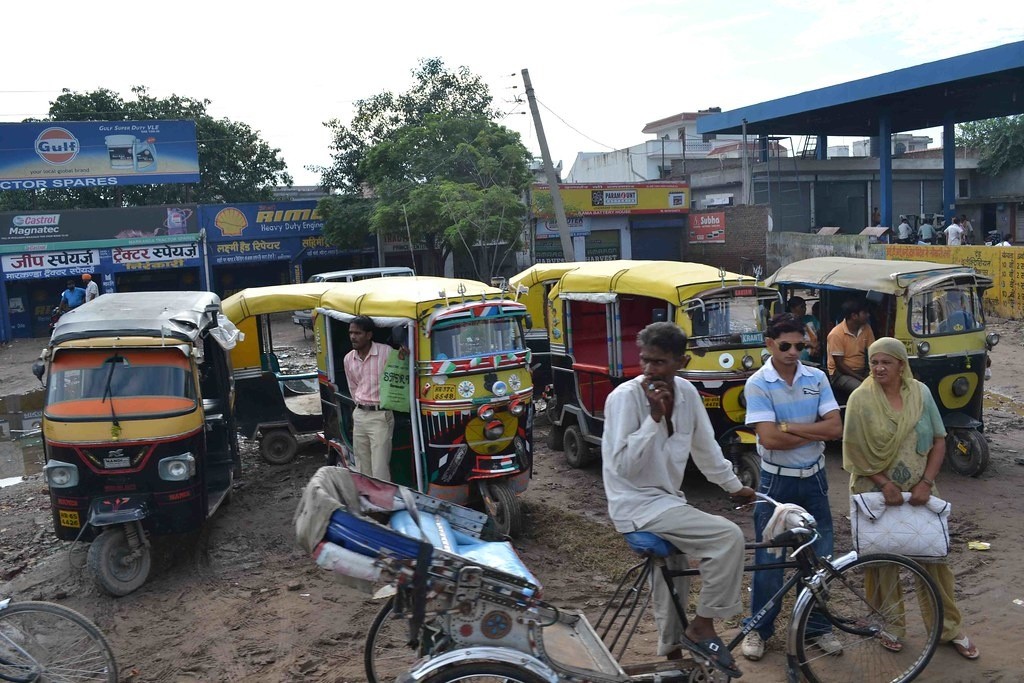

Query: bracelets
[878,479,890,489]
[923,476,934,486]
[781,421,787,431]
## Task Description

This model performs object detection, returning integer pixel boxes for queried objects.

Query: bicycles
[1,599,118,683]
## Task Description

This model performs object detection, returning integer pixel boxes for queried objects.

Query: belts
[357,403,389,412]
[760,453,826,480]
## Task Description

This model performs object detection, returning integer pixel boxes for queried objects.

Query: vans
[292,266,414,330]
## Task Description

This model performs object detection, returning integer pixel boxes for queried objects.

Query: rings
[649,384,656,390]
[654,389,661,393]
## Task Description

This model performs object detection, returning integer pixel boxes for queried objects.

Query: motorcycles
[221,282,343,465]
[313,274,548,544]
[550,259,792,499]
[497,261,594,404]
[32,291,244,597]
[761,257,1002,481]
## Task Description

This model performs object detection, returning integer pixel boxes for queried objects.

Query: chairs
[390,509,543,600]
[200,366,220,416]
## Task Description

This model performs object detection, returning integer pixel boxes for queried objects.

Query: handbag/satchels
[378,349,411,413]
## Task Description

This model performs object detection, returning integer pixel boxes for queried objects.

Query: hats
[81,274,92,280]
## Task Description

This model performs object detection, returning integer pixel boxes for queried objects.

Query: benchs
[566,338,646,412]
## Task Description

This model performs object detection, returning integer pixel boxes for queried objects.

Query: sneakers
[740,630,765,661]
[811,633,844,656]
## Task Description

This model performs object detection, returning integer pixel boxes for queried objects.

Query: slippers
[679,632,744,679]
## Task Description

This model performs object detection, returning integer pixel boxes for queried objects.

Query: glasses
[769,337,805,352]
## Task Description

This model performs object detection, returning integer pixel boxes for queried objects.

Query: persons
[812,301,838,334]
[601,322,757,678]
[743,313,843,660]
[82,273,99,303]
[344,315,395,484]
[894,215,915,244]
[787,297,821,363]
[995,234,1014,246]
[943,216,967,245]
[960,215,973,243]
[827,297,875,396]
[871,207,881,227]
[58,278,86,313]
[843,337,980,660]
[917,218,938,245]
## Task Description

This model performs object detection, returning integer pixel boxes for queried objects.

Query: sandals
[879,630,904,652]
[951,634,980,660]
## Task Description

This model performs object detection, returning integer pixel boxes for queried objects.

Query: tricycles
[291,465,944,683]
